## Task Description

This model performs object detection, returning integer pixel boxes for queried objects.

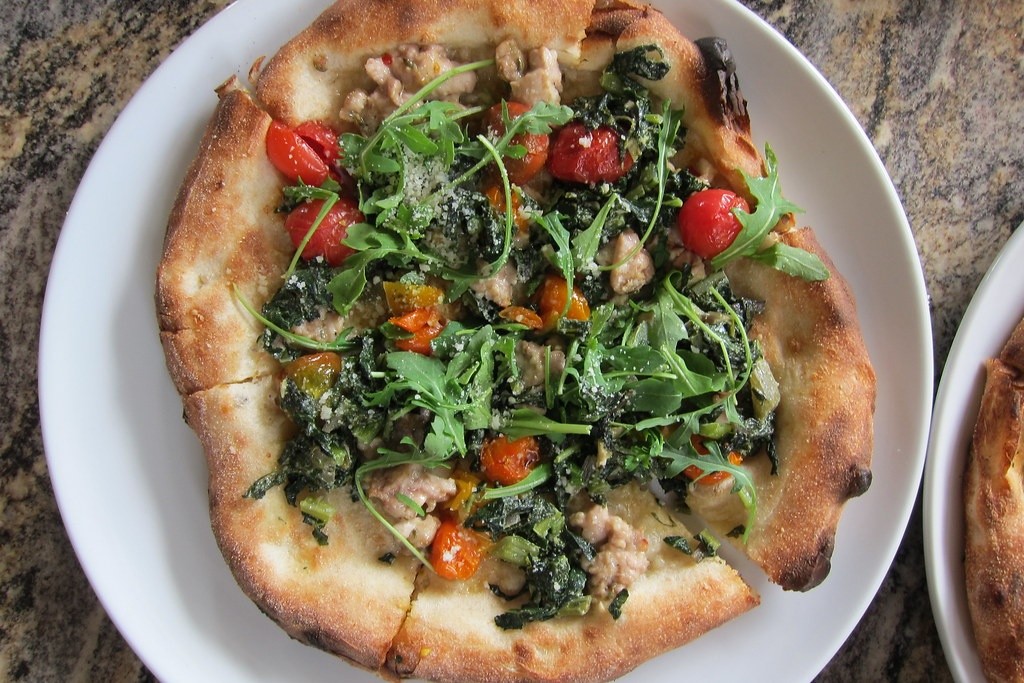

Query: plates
[37,0,934,683]
[922,216,1024,683]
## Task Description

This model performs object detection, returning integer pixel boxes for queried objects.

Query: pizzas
[153,0,876,683]
[959,310,1024,683]
